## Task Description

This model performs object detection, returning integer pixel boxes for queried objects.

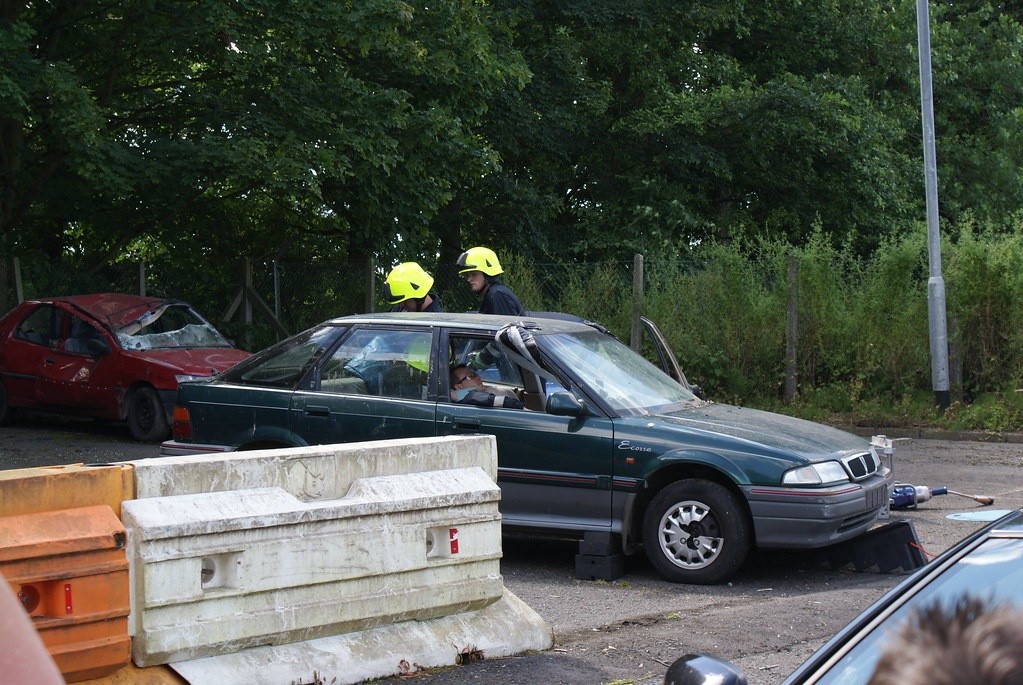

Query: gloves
[465,351,490,371]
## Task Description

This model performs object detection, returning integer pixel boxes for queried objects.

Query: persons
[383,262,453,401]
[450,364,525,409]
[866,584,1023,685]
[456,246,525,371]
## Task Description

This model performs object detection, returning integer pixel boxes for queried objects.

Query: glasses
[457,372,478,384]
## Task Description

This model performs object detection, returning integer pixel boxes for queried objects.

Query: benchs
[319,372,414,393]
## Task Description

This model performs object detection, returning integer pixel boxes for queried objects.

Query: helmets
[383,262,434,304]
[456,247,505,276]
[404,335,455,373]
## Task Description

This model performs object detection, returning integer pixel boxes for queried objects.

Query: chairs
[65,318,99,353]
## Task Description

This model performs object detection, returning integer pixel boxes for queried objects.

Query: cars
[0,292,256,444]
[665,504,1022,685]
[160,310,891,585]
